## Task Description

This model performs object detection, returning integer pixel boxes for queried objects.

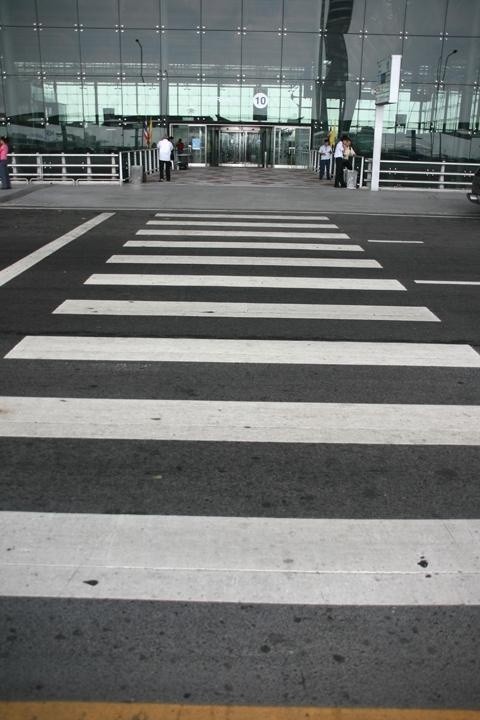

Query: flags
[144,116,150,148]
[149,119,153,143]
[328,123,336,147]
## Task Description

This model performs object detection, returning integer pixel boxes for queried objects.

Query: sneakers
[159,179,164,182]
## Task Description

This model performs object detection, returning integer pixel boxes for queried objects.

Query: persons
[177,137,186,166]
[168,136,175,170]
[333,135,348,188]
[317,139,334,179]
[156,133,175,182]
[342,138,356,170]
[1,135,12,191]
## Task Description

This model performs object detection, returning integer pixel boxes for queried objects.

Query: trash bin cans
[179,154,188,170]
[130,165,144,185]
[346,170,358,190]
